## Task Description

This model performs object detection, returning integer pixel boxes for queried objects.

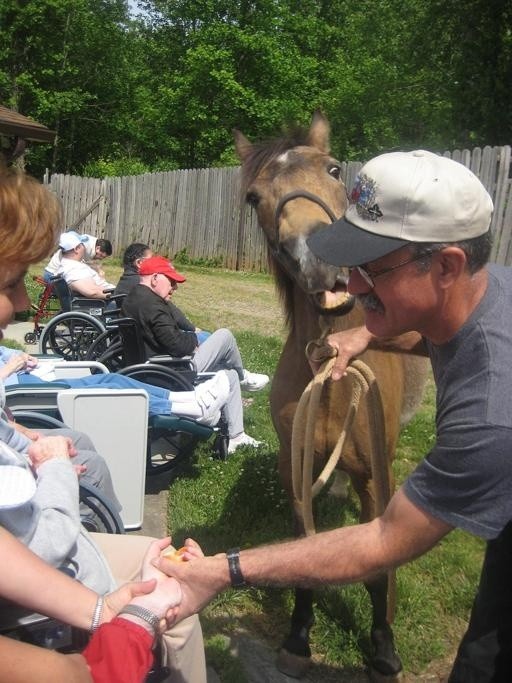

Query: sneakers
[239,368,270,394]
[226,430,266,455]
[193,369,230,428]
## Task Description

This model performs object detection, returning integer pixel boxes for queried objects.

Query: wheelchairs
[35,274,132,360]
[85,314,232,477]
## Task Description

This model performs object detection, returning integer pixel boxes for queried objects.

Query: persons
[112,241,210,355]
[0,167,206,680]
[121,254,272,393]
[55,227,117,302]
[150,144,511,682]
[43,230,112,284]
[0,406,123,532]
[0,373,208,683]
[1,106,59,330]
[0,346,268,455]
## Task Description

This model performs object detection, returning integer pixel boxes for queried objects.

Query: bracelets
[223,544,249,591]
[114,600,162,651]
[86,592,105,636]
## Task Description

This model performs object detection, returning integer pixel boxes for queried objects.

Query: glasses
[353,245,460,288]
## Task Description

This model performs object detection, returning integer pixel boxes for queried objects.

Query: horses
[230,107,430,683]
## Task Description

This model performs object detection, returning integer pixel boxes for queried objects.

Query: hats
[57,230,90,252]
[306,151,495,267]
[138,257,187,284]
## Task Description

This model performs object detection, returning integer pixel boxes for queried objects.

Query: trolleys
[23,273,61,345]
